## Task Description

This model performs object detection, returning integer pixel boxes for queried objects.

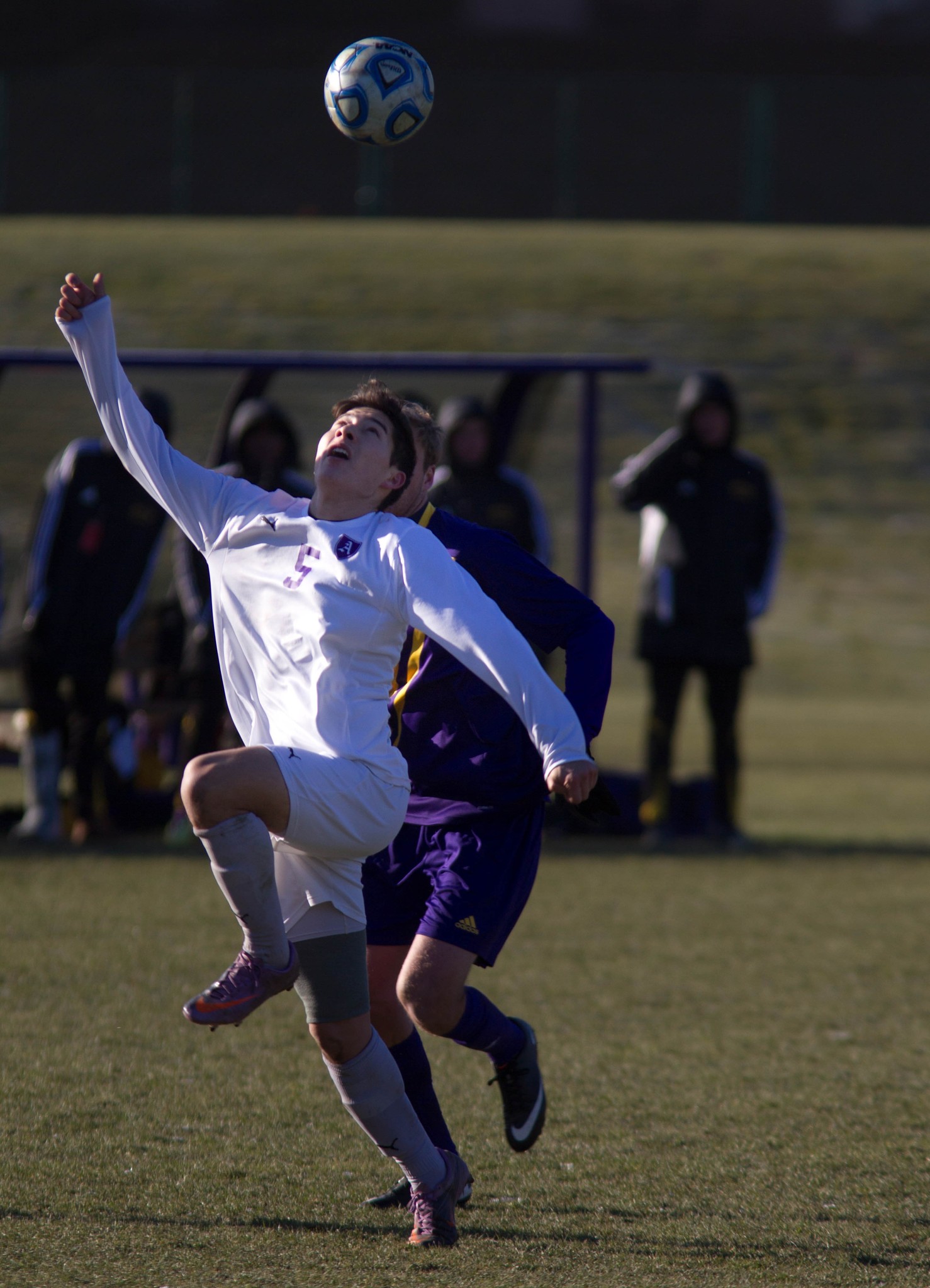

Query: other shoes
[160,817,198,849]
[639,778,670,825]
[11,809,59,842]
[72,818,102,847]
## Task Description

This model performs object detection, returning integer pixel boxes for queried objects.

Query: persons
[0,365,779,853]
[359,401,617,1214]
[50,269,602,1244]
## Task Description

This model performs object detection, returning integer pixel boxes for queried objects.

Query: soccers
[324,36,434,146]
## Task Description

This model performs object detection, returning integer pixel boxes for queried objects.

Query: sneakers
[486,1016,546,1152]
[183,941,299,1031]
[403,1145,470,1247]
[360,1173,473,1210]
